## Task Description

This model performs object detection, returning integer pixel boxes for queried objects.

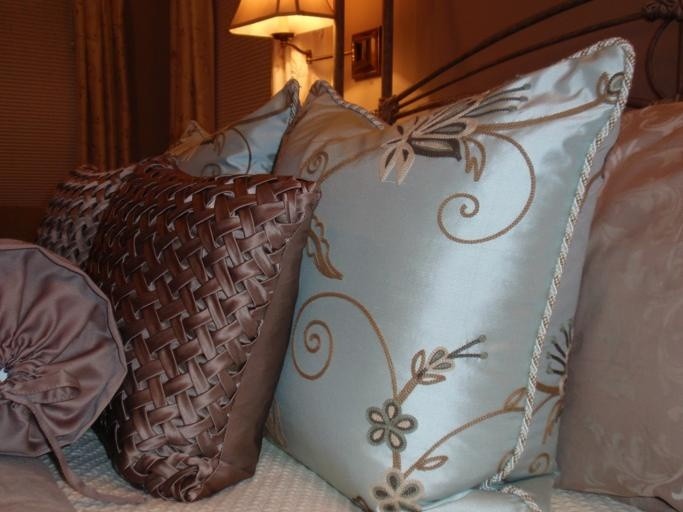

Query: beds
[0,0,683,512]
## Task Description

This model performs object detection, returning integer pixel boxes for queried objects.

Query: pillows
[161,77,301,176]
[260,36,637,512]
[84,160,321,501]
[555,99,683,512]
[0,241,128,459]
[34,162,137,266]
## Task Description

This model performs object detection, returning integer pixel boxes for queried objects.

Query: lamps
[229,0,383,81]
